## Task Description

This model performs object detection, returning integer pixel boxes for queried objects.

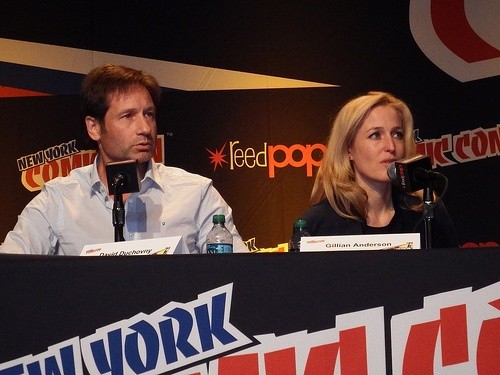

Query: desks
[1,246,500,375]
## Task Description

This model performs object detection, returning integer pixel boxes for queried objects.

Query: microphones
[105,156,142,194]
[386,152,442,193]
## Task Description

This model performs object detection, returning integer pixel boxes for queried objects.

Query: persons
[292,91,457,248]
[0,65,249,254]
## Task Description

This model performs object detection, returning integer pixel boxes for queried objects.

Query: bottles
[205,214,233,253]
[288,219,310,252]
